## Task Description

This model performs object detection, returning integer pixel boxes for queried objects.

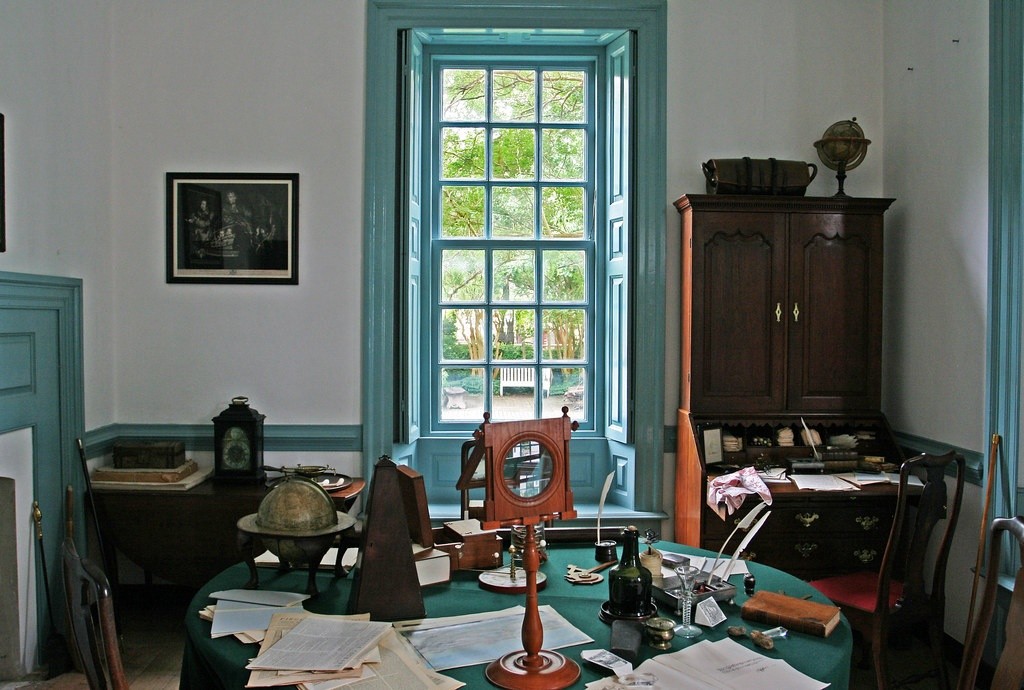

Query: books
[742,590,841,638]
[755,468,925,492]
[208,587,311,639]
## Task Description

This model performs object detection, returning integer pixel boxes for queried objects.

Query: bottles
[609,526,652,616]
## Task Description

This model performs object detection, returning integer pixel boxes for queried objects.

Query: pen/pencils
[707,499,769,585]
[597,470,615,545]
[800,417,820,462]
[720,511,771,583]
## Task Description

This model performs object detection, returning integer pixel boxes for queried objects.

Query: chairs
[55,540,130,690]
[954,514,1024,690]
[806,449,967,689]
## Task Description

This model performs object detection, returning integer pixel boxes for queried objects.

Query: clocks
[212,398,266,485]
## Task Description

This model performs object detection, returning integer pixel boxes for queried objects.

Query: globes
[236,475,364,613]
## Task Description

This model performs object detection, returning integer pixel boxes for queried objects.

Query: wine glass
[673,566,702,637]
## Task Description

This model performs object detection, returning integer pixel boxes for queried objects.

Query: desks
[178,537,850,690]
[675,409,951,554]
[88,474,367,637]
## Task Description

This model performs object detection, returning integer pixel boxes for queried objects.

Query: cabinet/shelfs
[673,191,895,411]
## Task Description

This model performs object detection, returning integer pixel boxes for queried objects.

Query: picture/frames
[165,171,303,285]
